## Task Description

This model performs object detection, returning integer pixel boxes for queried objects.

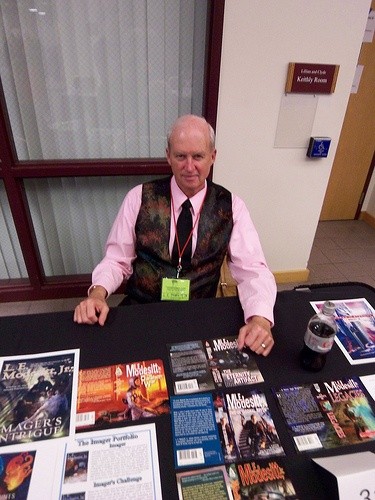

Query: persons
[125,376,158,420]
[73,114,278,357]
[216,414,278,459]
[13,375,69,437]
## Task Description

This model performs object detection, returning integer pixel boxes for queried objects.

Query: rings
[261,344,266,350]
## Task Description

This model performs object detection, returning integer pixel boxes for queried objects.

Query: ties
[171,200,193,263]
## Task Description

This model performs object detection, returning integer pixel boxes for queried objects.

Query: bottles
[302,302,336,373]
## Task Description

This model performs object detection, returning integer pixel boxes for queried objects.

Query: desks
[0,285,375,500]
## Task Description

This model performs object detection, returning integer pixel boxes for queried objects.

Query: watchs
[91,286,100,290]
